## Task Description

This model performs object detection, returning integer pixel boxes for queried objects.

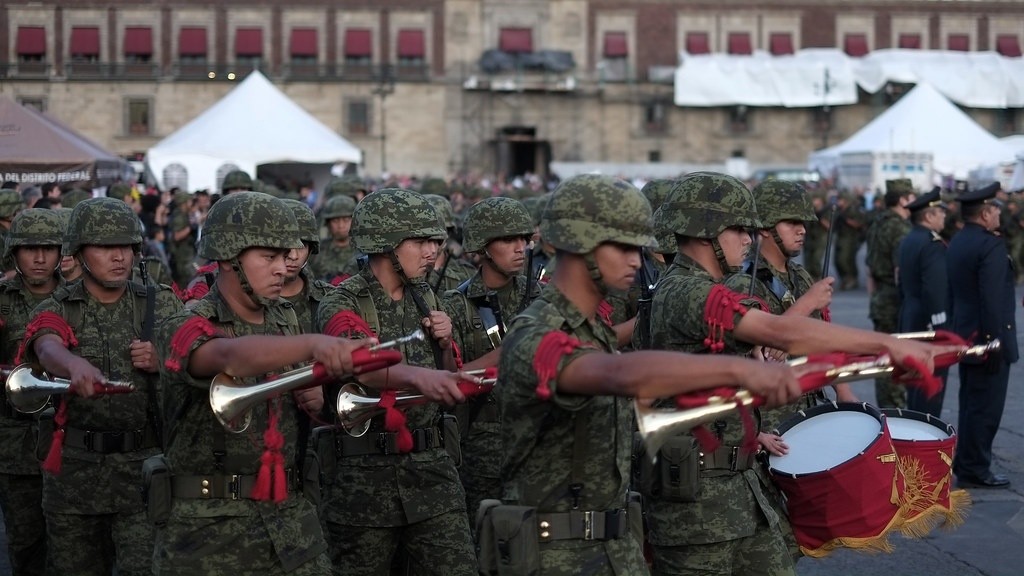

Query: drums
[759,400,908,560]
[877,406,957,528]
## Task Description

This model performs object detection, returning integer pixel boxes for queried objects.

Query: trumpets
[1,361,137,415]
[206,325,426,435]
[312,366,500,438]
[631,348,894,467]
[786,328,1002,382]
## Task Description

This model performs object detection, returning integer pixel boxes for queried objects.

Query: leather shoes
[957,471,1010,489]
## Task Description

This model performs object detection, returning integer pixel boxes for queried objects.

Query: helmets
[753,179,819,228]
[541,174,660,254]
[0,188,22,217]
[106,183,131,202]
[640,176,677,209]
[321,170,532,254]
[67,198,143,255]
[62,190,88,208]
[654,172,763,238]
[176,191,191,204]
[10,209,66,246]
[198,190,302,260]
[283,198,319,242]
[222,171,254,193]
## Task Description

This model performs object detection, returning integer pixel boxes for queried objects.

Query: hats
[885,180,917,194]
[903,187,948,212]
[954,181,1004,212]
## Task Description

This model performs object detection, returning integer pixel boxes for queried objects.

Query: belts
[16,375,826,537]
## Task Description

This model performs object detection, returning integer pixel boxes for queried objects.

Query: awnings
[342,29,426,57]
[686,31,793,55]
[501,30,530,52]
[15,26,208,57]
[900,34,1021,55]
[605,35,627,58]
[235,28,319,58]
[845,34,868,55]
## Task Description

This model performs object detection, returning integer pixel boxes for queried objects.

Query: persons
[0,167,1024,575]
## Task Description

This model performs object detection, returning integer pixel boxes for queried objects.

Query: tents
[807,83,1017,196]
[146,69,360,190]
[0,92,134,190]
